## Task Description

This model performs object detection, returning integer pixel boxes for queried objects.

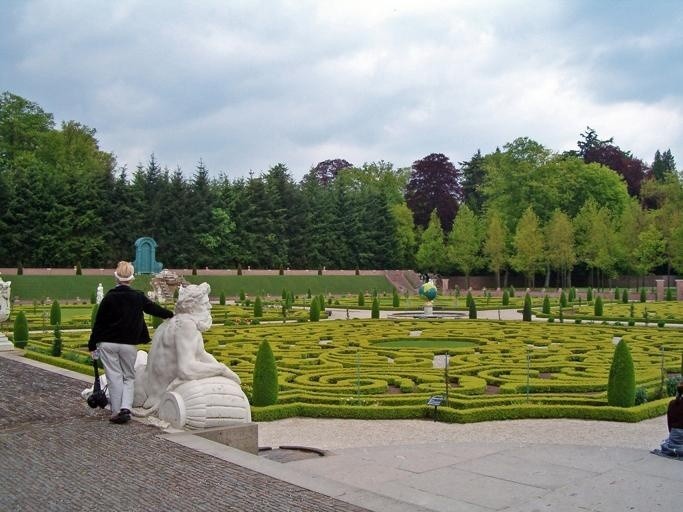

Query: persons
[666,382,683,434]
[85,259,176,424]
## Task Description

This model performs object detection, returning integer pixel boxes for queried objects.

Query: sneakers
[110,410,130,424]
